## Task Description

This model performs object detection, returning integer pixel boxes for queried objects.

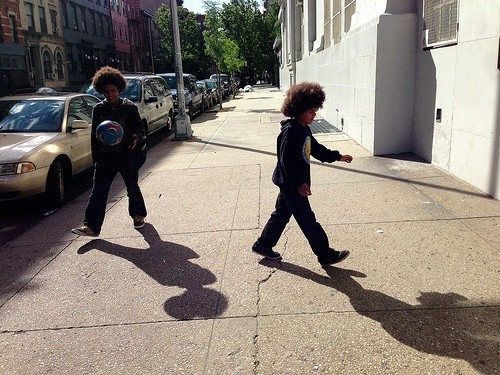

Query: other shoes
[134,215,145,229]
[71,225,99,237]
[321,250,351,266]
[252,243,282,259]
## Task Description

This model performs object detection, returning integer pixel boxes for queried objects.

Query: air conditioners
[48,73,57,79]
[53,27,60,32]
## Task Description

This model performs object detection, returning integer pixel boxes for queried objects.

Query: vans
[85,74,175,150]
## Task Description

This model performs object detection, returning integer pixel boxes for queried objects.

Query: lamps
[93,56,97,60]
[110,58,119,63]
[85,54,90,59]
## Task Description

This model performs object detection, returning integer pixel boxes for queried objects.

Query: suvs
[154,73,204,121]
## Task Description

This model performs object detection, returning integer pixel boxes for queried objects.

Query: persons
[70,66,147,237]
[252,82,353,268]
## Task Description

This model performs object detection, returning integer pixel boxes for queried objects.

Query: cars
[196,73,232,110]
[0,90,106,209]
